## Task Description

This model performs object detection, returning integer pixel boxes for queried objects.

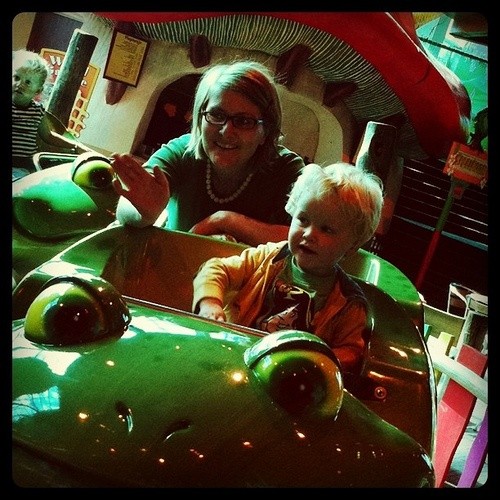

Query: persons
[192,161,384,371]
[12,49,75,173]
[113,62,305,248]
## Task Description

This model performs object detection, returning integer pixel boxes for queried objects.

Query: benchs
[112,225,252,315]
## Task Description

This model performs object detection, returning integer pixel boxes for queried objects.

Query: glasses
[201,110,265,130]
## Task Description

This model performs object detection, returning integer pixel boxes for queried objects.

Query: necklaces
[207,159,253,203]
